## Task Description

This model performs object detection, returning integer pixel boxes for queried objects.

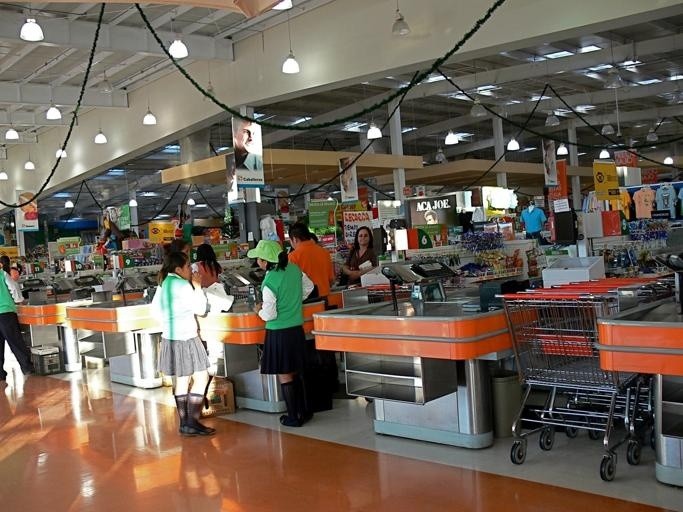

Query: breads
[19,192,37,212]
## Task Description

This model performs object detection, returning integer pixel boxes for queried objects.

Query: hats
[247,240,283,264]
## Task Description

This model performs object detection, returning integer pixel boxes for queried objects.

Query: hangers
[641,181,670,190]
[528,201,534,212]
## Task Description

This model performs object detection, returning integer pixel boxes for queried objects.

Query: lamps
[65,199,74,208]
[599,109,610,159]
[46,101,62,119]
[19,3,44,42]
[469,72,488,118]
[143,110,157,126]
[601,77,615,135]
[544,86,560,127]
[129,197,138,207]
[557,110,568,156]
[168,40,188,59]
[187,197,195,206]
[0,167,8,180]
[646,89,659,141]
[24,153,35,170]
[368,89,383,140]
[435,137,446,161]
[98,71,113,95]
[443,88,459,145]
[390,1,411,37]
[281,11,301,74]
[4,122,19,140]
[55,143,68,158]
[663,127,673,165]
[95,130,108,144]
[506,104,520,152]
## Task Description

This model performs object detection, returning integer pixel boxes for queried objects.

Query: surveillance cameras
[617,132,623,138]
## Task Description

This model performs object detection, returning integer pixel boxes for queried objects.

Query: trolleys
[495,275,676,481]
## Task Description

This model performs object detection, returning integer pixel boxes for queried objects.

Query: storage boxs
[541,255,606,289]
[198,378,236,419]
[29,344,66,375]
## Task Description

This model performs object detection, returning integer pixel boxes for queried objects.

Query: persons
[189,243,224,287]
[179,204,190,229]
[340,226,377,282]
[424,209,438,224]
[232,118,264,173]
[519,200,548,245]
[247,222,352,425]
[459,208,476,234]
[277,191,290,213]
[167,239,189,257]
[0,256,35,381]
[19,192,38,221]
[149,251,216,437]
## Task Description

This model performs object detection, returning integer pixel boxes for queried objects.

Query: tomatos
[25,212,37,220]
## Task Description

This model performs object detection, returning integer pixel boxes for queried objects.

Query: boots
[279,381,305,427]
[175,393,216,436]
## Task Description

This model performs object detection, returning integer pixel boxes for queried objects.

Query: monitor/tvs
[553,208,578,245]
[373,228,386,255]
